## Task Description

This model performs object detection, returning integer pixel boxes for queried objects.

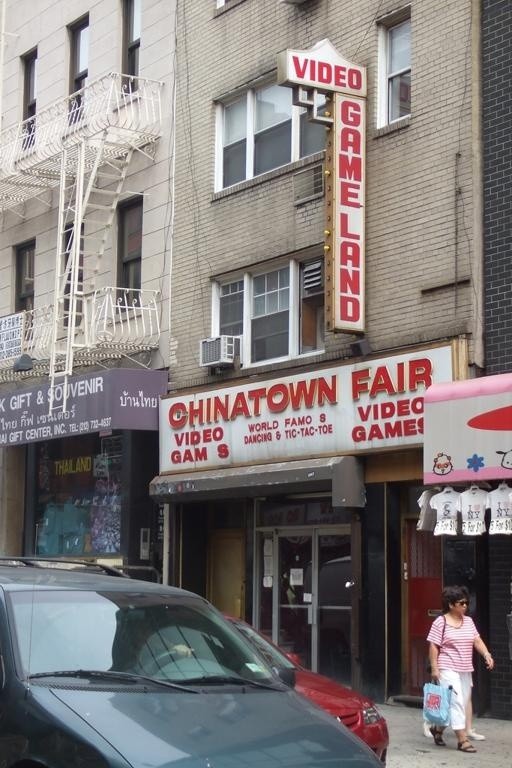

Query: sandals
[430,726,445,745]
[457,740,476,752]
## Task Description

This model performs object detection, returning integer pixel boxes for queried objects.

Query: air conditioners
[196,334,239,375]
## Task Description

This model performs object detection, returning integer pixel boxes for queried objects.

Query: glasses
[456,601,469,605]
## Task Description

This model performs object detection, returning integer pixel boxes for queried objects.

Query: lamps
[12,352,37,373]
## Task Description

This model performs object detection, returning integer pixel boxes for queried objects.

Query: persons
[422,585,494,754]
[125,617,195,658]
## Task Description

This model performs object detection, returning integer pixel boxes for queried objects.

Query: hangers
[432,483,511,492]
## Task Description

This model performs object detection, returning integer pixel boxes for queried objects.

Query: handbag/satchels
[422,683,451,726]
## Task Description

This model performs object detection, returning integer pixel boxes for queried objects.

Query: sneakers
[465,728,485,741]
[423,722,434,737]
